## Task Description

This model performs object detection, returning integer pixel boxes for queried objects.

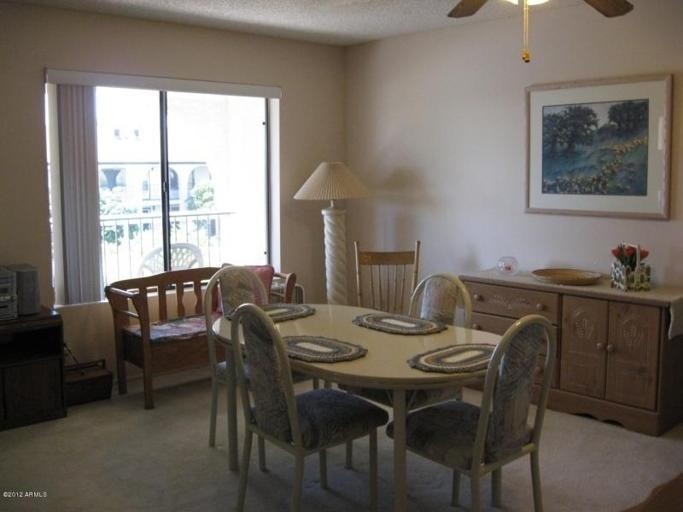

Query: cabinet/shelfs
[458,278,561,394]
[559,291,682,414]
[0,305,63,432]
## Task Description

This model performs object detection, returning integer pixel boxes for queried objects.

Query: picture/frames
[524,72,672,222]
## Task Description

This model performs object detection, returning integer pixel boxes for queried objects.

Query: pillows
[221,262,273,300]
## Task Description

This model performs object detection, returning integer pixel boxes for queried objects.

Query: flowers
[611,246,648,263]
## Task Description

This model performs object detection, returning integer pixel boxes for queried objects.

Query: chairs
[386,313,560,511]
[335,272,474,470]
[352,239,421,322]
[204,266,319,472]
[230,300,388,510]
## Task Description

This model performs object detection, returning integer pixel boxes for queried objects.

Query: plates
[530,268,604,285]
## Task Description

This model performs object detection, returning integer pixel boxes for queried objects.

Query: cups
[495,257,519,275]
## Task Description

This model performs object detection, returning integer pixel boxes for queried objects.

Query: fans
[446,0,634,20]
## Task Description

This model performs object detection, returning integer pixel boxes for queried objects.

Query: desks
[210,303,507,511]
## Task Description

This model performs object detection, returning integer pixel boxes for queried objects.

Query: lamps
[505,0,549,64]
[290,161,377,305]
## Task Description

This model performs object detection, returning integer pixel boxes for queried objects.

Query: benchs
[104,269,297,410]
